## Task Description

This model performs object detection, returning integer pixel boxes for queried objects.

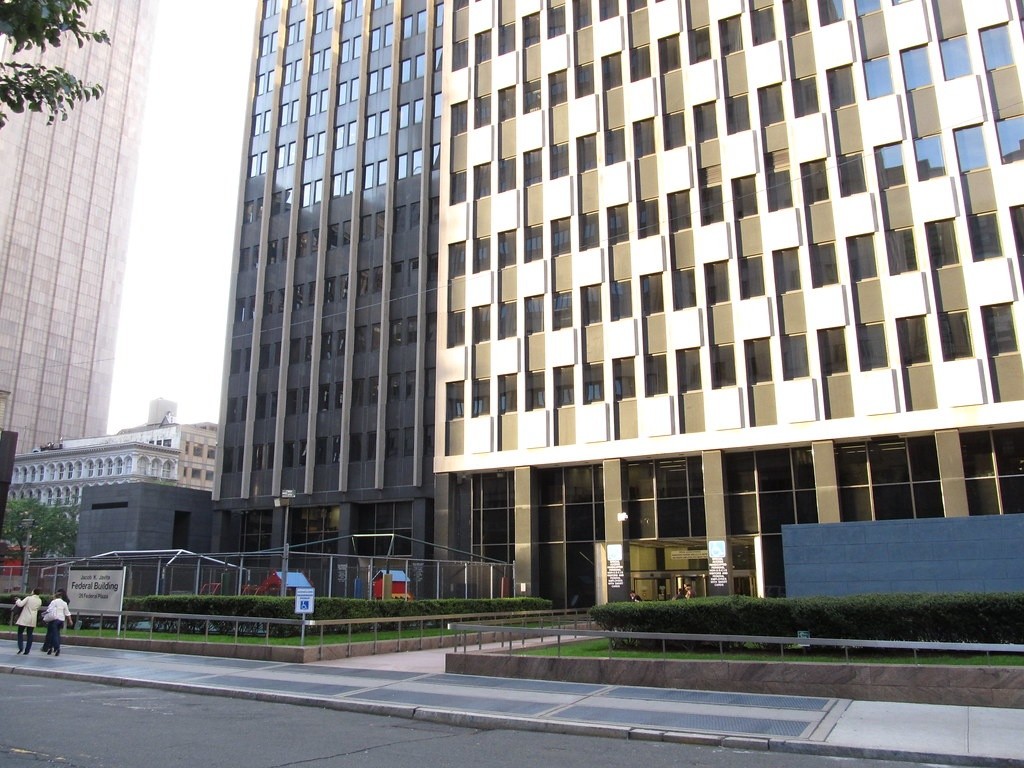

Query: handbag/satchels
[43,600,58,622]
[11,597,25,615]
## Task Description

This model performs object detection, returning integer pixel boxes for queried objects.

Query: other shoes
[54,649,60,656]
[24,651,29,655]
[47,649,52,655]
[17,649,23,654]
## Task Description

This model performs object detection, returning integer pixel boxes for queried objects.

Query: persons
[684,583,695,599]
[14,588,42,655]
[676,588,685,599]
[629,591,642,601]
[40,589,74,657]
